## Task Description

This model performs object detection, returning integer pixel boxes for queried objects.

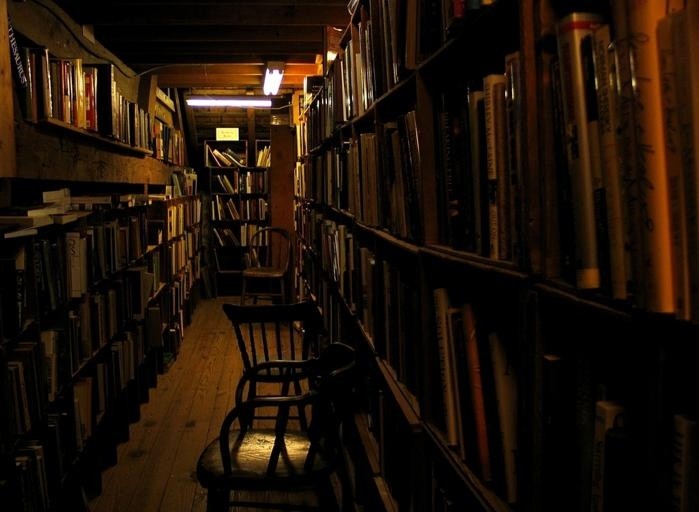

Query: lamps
[263,60,285,96]
[184,93,273,108]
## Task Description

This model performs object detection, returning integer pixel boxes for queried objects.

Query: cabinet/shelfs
[205,139,271,297]
[0,0,202,512]
[293,0,699,511]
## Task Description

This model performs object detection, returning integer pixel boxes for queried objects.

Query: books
[1,18,201,512]
[288,1,699,512]
[204,143,273,268]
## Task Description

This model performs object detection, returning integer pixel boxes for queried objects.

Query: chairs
[242,225,293,326]
[193,300,359,512]
